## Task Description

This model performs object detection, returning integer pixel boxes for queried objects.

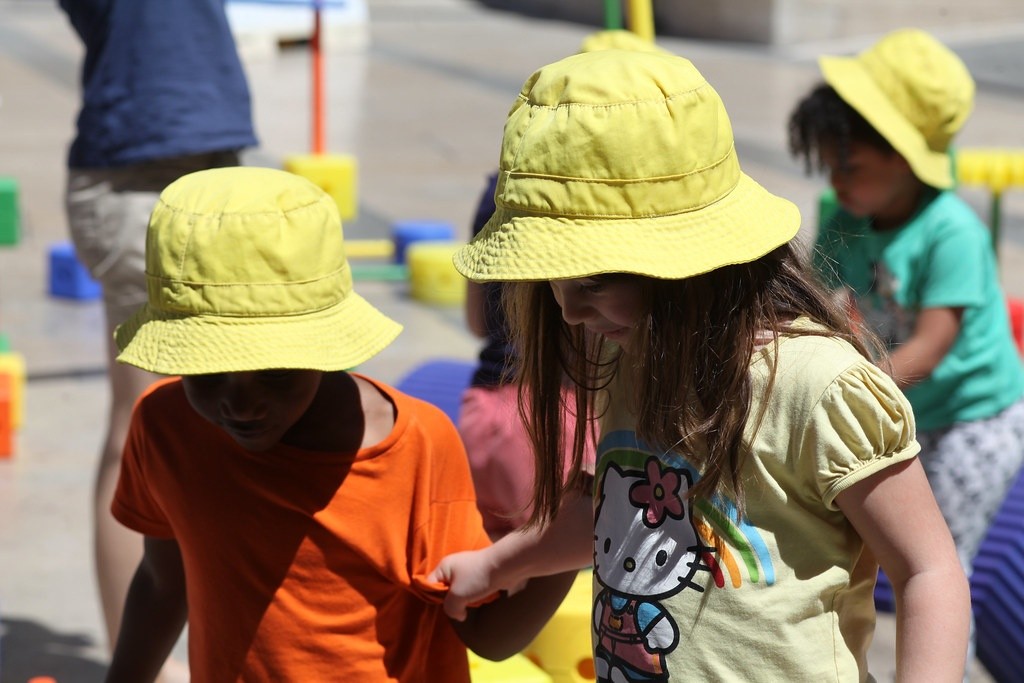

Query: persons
[781,23,1023,590]
[105,165,598,682]
[449,165,598,682]
[45,0,262,657]
[449,32,977,682]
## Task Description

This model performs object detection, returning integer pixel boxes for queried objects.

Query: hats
[452,48,801,284]
[816,28,978,187]
[112,167,404,377]
